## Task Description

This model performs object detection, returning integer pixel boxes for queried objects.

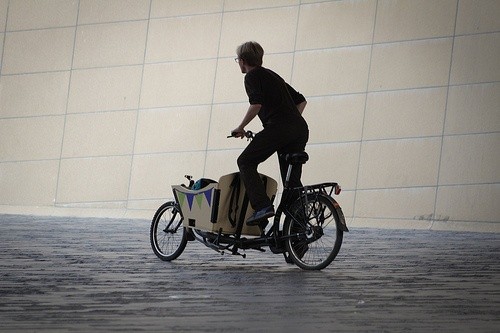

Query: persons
[235,41,309,264]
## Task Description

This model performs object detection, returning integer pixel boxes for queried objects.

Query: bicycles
[146,130,348,269]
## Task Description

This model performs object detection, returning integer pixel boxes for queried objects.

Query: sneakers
[247,206,275,224]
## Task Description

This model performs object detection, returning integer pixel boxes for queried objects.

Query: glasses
[235,58,241,63]
